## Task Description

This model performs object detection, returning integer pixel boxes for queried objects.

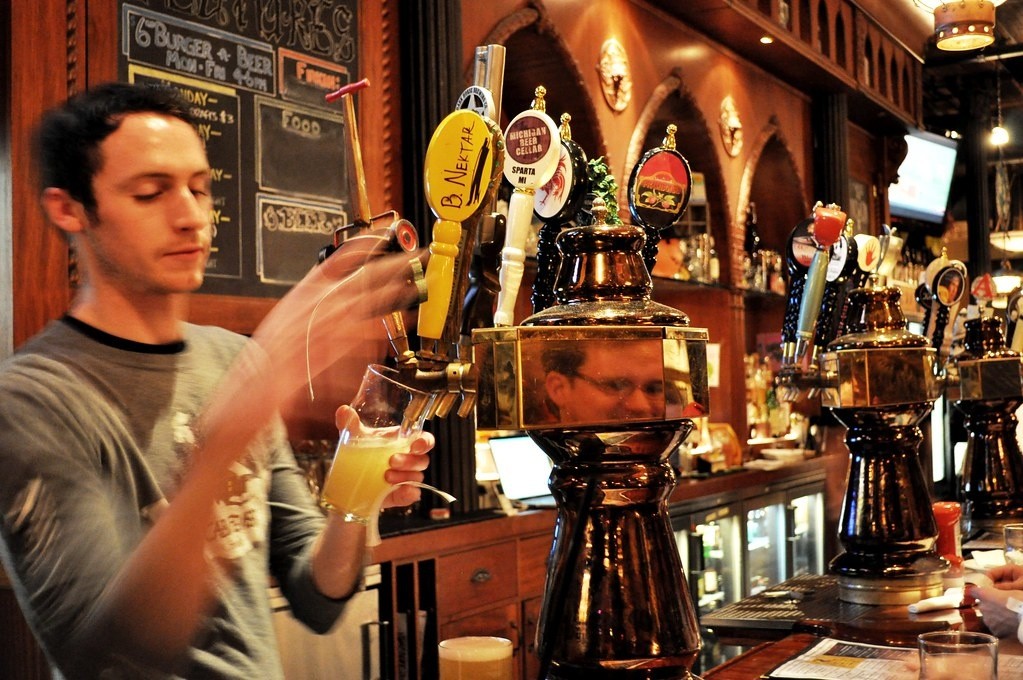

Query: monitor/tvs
[886,126,957,225]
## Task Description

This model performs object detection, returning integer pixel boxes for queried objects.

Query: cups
[318,365,439,528]
[918,631,1000,680]
[437,635,513,680]
[1003,523,1023,570]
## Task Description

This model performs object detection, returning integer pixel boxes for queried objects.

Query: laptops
[488,434,557,509]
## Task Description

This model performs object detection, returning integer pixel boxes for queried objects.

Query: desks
[701,519,1023,679]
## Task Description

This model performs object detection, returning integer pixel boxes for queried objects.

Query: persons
[1,82,435,680]
[946,275,960,302]
[970,565,1023,644]
[543,346,709,422]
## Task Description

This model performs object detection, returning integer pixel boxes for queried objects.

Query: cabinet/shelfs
[389,455,845,680]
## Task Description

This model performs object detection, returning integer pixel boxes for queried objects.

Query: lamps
[933,1,996,50]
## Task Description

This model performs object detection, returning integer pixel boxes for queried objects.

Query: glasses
[557,366,665,400]
